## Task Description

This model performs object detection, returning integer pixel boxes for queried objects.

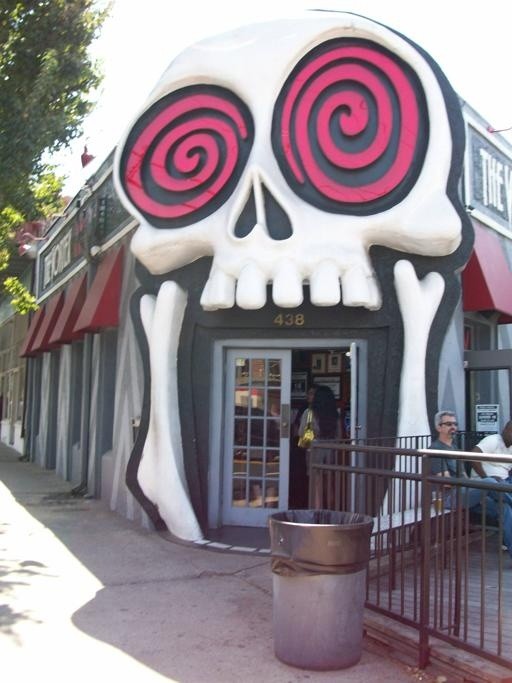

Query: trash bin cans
[268,510,374,671]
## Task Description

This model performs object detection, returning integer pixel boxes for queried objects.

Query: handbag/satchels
[298,428,314,448]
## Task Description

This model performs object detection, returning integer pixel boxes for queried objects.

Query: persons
[467,419,511,550]
[290,383,317,507]
[298,387,344,509]
[425,409,512,557]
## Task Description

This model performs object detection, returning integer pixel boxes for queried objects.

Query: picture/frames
[290,350,350,438]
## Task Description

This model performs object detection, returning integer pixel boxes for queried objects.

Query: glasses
[441,421,459,425]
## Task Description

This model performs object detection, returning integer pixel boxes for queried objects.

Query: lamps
[81,136,118,168]
[18,230,49,256]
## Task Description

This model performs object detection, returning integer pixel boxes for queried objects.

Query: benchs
[369,501,482,587]
